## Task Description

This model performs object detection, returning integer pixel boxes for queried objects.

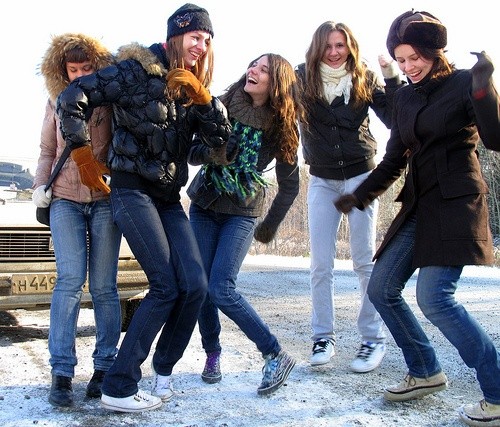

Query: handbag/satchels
[36,208,49,226]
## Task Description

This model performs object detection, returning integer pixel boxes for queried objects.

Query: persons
[332,8,500,427]
[187,54,312,395]
[32,32,122,406]
[293,21,406,373]
[57,3,232,412]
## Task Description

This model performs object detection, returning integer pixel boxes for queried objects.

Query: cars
[0,161,149,331]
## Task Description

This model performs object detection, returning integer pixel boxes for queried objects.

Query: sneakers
[350,342,386,372]
[202,353,222,383]
[257,348,296,395]
[151,358,175,401]
[460,399,500,425]
[311,338,335,364]
[100,389,162,412]
[384,370,448,399]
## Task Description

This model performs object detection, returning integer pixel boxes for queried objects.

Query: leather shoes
[49,374,74,407]
[87,369,109,396]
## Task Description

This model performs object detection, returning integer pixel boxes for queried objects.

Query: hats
[65,47,91,64]
[386,10,447,60]
[166,3,214,40]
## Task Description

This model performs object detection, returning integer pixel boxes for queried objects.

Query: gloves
[71,145,112,194]
[253,218,279,244]
[333,194,359,214]
[165,68,212,105]
[31,185,52,208]
[471,51,494,93]
